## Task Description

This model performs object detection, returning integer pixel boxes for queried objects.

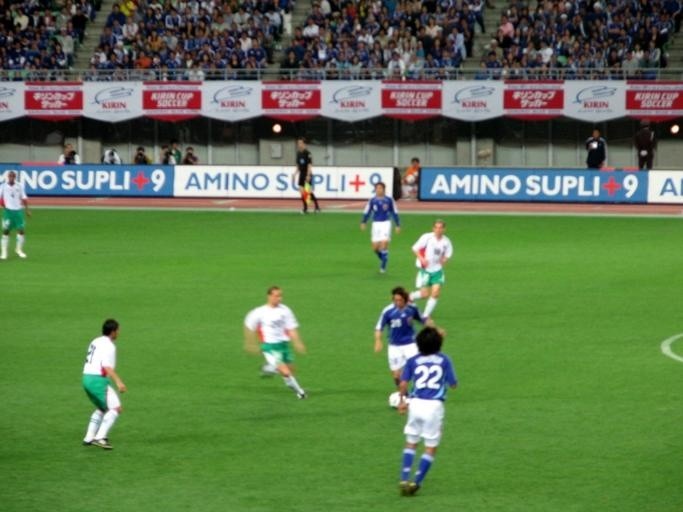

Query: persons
[360,182,400,273]
[183,147,197,165]
[0,171,31,259]
[242,286,307,399]
[293,136,319,211]
[58,143,80,165]
[400,157,421,197]
[585,128,609,170]
[399,327,458,496]
[408,220,454,319]
[1,1,683,81]
[375,287,446,396]
[633,118,658,170]
[100,147,152,165]
[81,319,127,449]
[160,139,181,165]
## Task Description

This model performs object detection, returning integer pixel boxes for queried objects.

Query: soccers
[388,391,406,408]
[406,175,416,185]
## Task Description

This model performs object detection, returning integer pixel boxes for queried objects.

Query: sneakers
[296,206,321,215]
[295,390,308,401]
[82,434,113,452]
[378,256,389,274]
[394,480,421,498]
[256,365,272,378]
[1,248,30,260]
